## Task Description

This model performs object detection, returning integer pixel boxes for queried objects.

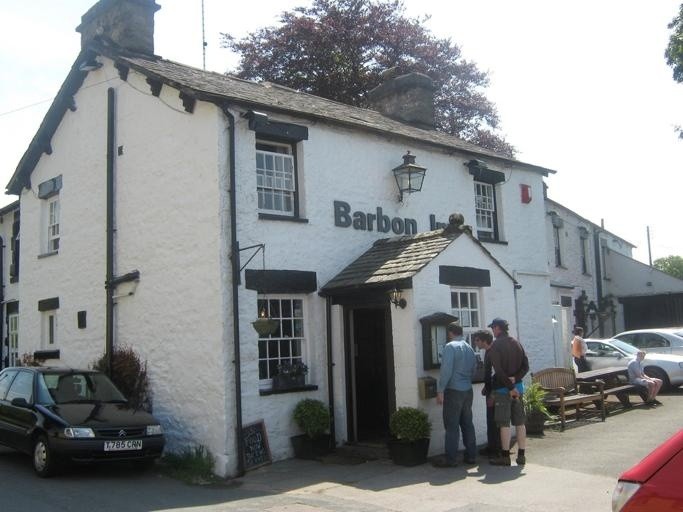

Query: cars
[613,326,683,356]
[571,337,683,395]
[0,366,165,480]
[612,428,683,512]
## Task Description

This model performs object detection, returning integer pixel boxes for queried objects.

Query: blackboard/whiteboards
[235,418,272,472]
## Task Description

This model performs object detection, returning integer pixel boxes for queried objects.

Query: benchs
[573,366,648,422]
[527,365,607,432]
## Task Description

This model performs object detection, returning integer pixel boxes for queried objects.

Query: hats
[488,317,509,329]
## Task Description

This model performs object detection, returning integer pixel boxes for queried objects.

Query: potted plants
[520,381,554,436]
[387,405,432,467]
[287,397,334,461]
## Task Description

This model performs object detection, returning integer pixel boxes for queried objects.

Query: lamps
[389,149,430,204]
[77,57,104,73]
[384,285,408,312]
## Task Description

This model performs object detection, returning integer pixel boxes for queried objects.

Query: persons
[431,319,477,467]
[472,329,503,455]
[629,350,663,405]
[570,326,595,397]
[487,317,528,465]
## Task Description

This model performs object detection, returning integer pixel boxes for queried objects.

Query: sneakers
[516,457,525,464]
[432,460,458,468]
[464,456,476,464]
[646,399,662,408]
[480,446,511,466]
